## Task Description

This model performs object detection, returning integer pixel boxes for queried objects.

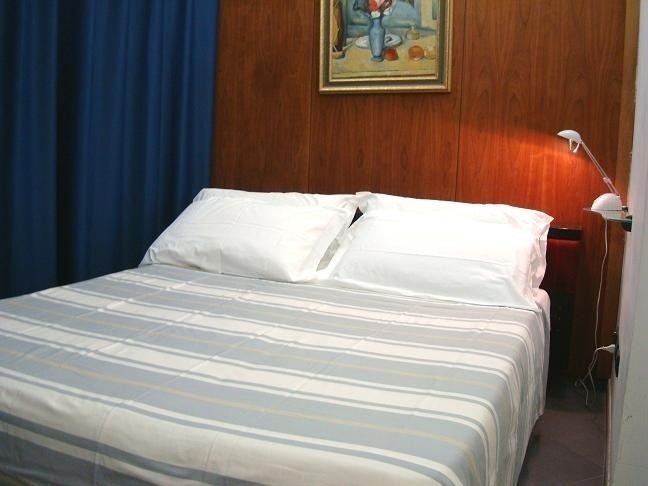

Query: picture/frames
[317,0,454,94]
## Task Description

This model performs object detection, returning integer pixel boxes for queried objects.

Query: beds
[0,225,582,486]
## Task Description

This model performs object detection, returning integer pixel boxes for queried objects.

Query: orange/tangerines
[408,45,424,61]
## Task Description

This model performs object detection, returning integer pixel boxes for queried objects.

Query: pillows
[137,186,364,285]
[317,191,555,314]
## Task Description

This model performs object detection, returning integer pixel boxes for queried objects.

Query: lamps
[557,130,623,210]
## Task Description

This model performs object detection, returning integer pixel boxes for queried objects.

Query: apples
[383,48,398,60]
